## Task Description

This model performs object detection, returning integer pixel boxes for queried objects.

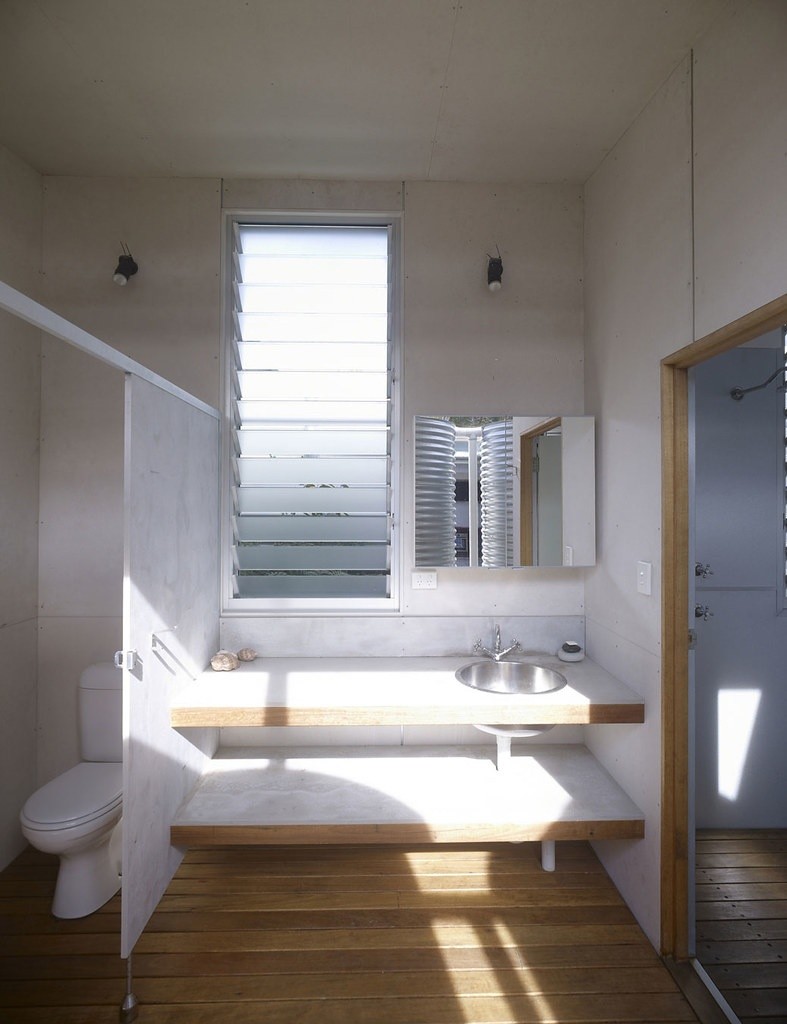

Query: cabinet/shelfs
[170,655,645,872]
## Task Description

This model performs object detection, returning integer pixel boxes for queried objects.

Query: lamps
[112,240,138,286]
[484,244,504,292]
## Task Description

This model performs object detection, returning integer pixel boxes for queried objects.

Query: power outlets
[412,570,437,590]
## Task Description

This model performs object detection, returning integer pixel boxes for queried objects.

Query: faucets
[493,624,502,661]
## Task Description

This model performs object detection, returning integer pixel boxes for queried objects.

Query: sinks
[455,662,567,695]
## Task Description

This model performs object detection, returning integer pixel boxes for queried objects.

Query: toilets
[21,662,121,918]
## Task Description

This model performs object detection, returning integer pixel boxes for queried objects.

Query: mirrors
[414,414,597,567]
